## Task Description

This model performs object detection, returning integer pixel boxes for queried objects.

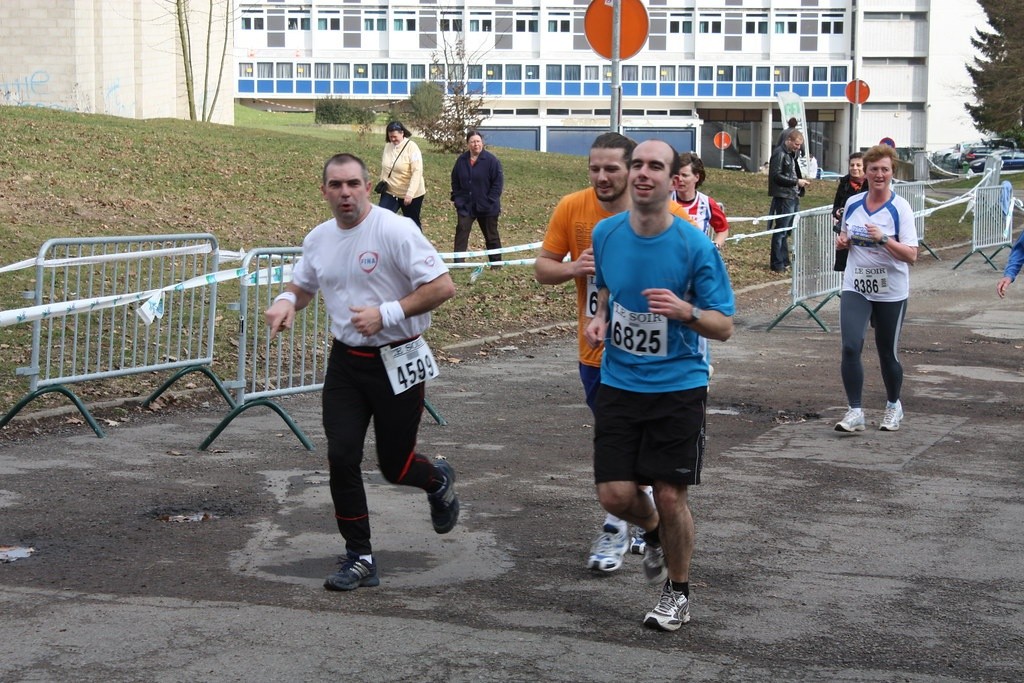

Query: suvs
[934,138,1018,169]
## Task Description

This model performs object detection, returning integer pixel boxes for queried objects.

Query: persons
[582,140,735,632]
[833,152,869,271]
[449,131,502,270]
[997,229,1024,299]
[265,153,461,589]
[776,118,805,159]
[672,152,730,250]
[761,162,769,175]
[534,132,698,571]
[810,153,817,179]
[768,130,810,273]
[378,121,427,230]
[834,143,919,432]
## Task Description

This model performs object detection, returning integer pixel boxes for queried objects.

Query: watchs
[685,307,701,324]
[879,234,888,245]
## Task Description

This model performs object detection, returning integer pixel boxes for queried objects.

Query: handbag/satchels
[374,180,388,195]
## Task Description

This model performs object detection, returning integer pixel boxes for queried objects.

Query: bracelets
[714,243,721,250]
[380,300,405,329]
[274,292,296,304]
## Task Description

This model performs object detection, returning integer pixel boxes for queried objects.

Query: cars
[970,149,1024,173]
[822,171,844,182]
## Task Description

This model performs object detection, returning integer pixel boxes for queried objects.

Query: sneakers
[878,399,904,431]
[629,525,652,562]
[323,549,380,591]
[587,522,630,573]
[426,459,459,534]
[643,580,691,632]
[641,538,669,586]
[834,404,866,432]
